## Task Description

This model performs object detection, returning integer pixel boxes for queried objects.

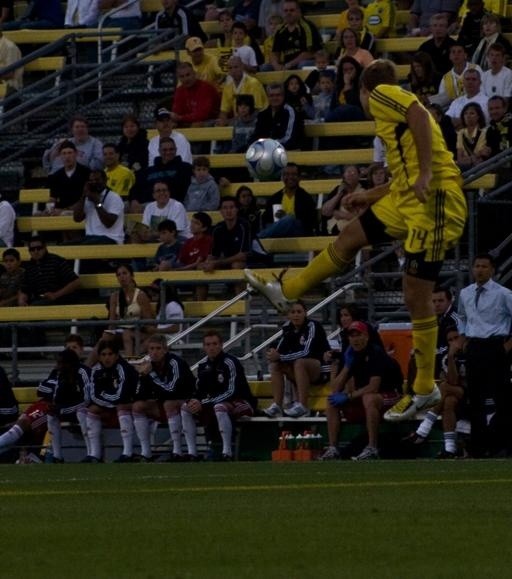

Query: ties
[474,286,484,308]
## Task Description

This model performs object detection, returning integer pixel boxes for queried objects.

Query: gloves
[328,392,348,408]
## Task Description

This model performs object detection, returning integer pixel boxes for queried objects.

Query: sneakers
[54,457,65,464]
[113,454,133,463]
[383,383,442,422]
[214,454,233,462]
[357,447,378,461]
[241,267,297,314]
[182,454,198,462]
[80,455,100,463]
[318,447,339,461]
[134,455,154,463]
[160,453,181,462]
[283,403,311,418]
[262,403,283,418]
[403,432,425,448]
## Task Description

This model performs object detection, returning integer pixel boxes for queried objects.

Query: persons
[320,320,402,461]
[32,140,93,243]
[252,161,316,269]
[366,166,389,187]
[159,213,213,302]
[247,82,303,150]
[261,1,320,70]
[86,340,139,462]
[236,186,260,234]
[80,262,155,367]
[406,53,439,98]
[243,59,468,420]
[66,1,98,31]
[424,46,484,107]
[216,11,234,48]
[104,2,143,33]
[183,330,254,462]
[404,288,467,397]
[446,254,512,457]
[183,157,220,212]
[337,8,373,54]
[12,237,80,358]
[1,32,23,115]
[97,143,134,202]
[408,323,471,460]
[227,94,261,153]
[481,96,512,158]
[456,103,493,167]
[431,104,457,157]
[323,56,372,150]
[264,298,330,415]
[362,0,393,62]
[158,62,218,155]
[456,1,507,35]
[227,25,258,81]
[469,13,512,68]
[279,75,313,120]
[147,108,192,165]
[234,0,260,22]
[0,336,85,446]
[335,0,365,33]
[330,303,383,393]
[151,0,205,89]
[126,138,230,203]
[311,77,339,122]
[64,170,124,276]
[135,336,193,464]
[397,0,454,65]
[194,195,250,300]
[140,220,185,300]
[476,44,512,99]
[459,0,497,62]
[125,278,186,363]
[335,28,372,67]
[260,4,280,41]
[43,117,103,174]
[43,349,92,466]
[116,116,147,169]
[122,180,191,272]
[407,16,456,87]
[0,247,28,307]
[319,161,370,236]
[208,56,269,155]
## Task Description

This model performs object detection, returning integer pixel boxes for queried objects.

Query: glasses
[349,17,361,20]
[29,246,45,252]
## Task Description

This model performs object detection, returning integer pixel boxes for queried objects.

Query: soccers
[246,139,288,178]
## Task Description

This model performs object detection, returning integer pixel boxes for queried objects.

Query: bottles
[45,439,53,462]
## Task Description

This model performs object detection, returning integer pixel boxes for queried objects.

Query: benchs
[10,377,445,428]
[0,1,512,317]
[0,299,248,357]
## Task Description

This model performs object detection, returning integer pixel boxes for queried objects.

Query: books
[126,220,150,244]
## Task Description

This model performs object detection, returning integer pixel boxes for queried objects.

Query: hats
[186,37,204,51]
[347,321,368,336]
[155,108,171,119]
